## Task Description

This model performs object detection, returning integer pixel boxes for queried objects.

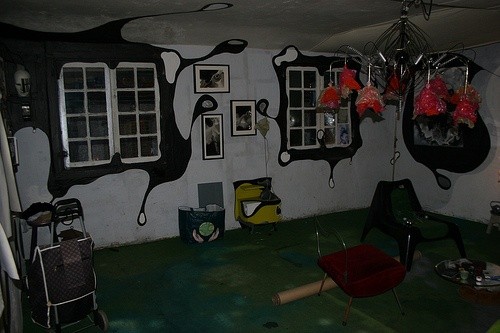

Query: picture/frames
[201,114,224,160]
[192,64,230,93]
[230,99,257,136]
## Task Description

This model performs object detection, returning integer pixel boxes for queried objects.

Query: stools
[27,214,51,260]
[49,198,86,245]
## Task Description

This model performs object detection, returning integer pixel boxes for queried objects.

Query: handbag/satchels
[178,203,226,242]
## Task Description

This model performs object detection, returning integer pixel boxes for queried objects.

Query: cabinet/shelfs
[3,41,49,131]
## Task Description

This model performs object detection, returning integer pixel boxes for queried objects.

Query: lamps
[257,117,274,200]
[320,0,478,127]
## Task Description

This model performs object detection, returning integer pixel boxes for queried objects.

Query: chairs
[361,178,465,272]
[313,213,405,325]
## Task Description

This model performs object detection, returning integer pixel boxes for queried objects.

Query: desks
[435,255,500,288]
